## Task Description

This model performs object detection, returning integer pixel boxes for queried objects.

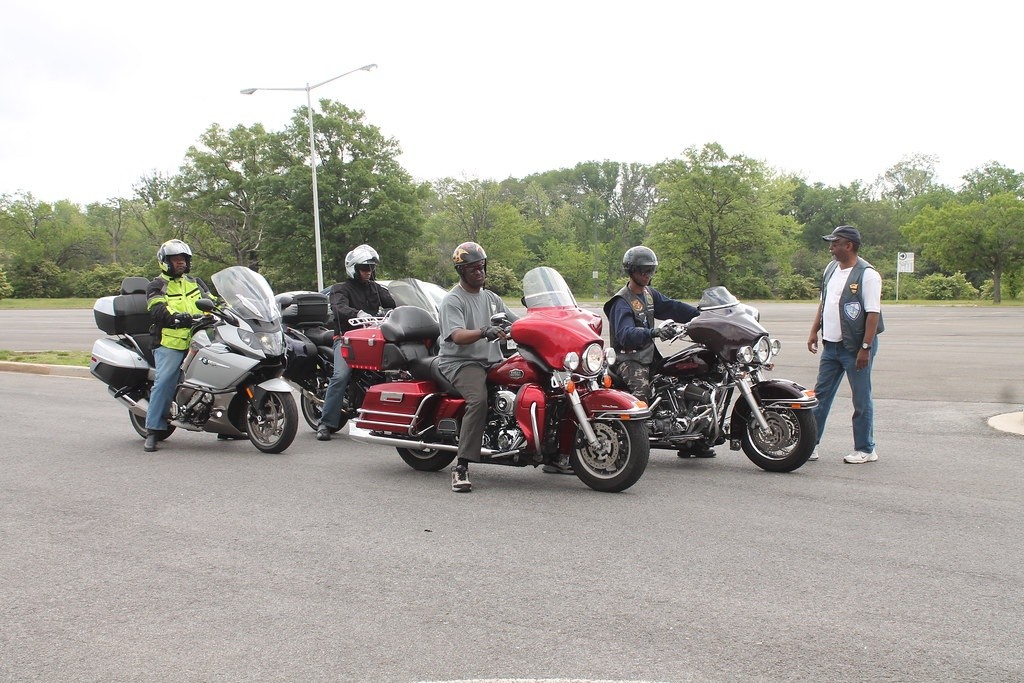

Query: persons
[807,225,885,463]
[438,242,576,491]
[317,245,397,441]
[144,239,249,451]
[604,246,716,458]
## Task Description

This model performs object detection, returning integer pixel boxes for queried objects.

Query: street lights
[238,61,379,291]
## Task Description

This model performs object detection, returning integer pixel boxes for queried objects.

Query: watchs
[861,342,872,350]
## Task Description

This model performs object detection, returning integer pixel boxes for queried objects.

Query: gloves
[357,311,373,327]
[650,323,677,340]
[173,312,193,329]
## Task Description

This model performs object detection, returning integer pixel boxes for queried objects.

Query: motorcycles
[269,288,397,433]
[598,284,818,473]
[331,265,656,493]
[88,264,299,455]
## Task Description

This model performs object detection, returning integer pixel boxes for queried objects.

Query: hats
[821,225,861,244]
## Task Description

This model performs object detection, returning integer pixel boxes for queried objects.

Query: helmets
[452,241,487,267]
[345,250,376,279]
[623,244,659,266]
[156,239,193,275]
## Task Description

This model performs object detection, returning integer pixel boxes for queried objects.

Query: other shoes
[677,446,717,459]
[317,422,330,440]
[218,431,249,440]
[144,435,157,451]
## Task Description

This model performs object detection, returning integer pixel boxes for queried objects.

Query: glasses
[356,264,375,272]
[631,267,655,275]
[459,263,485,274]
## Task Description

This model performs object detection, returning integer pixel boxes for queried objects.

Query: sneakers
[451,465,472,493]
[542,457,576,475]
[809,444,819,460]
[843,448,879,464]
